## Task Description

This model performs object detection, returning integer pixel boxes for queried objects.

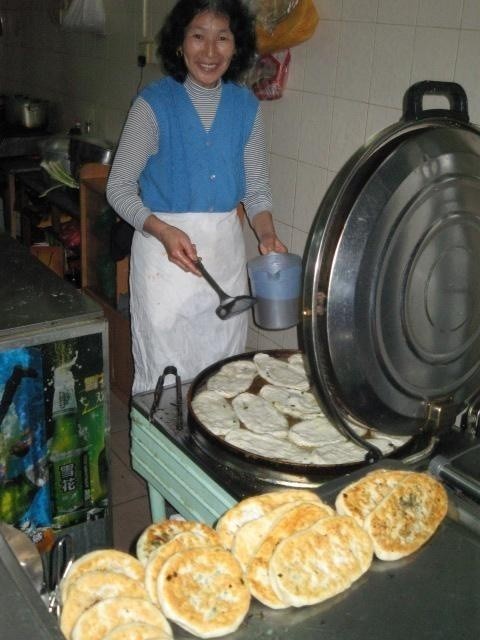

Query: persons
[101,2,292,401]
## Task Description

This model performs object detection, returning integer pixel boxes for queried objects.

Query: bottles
[0,368,90,530]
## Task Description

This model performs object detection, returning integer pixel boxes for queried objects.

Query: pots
[8,96,49,133]
[67,137,117,182]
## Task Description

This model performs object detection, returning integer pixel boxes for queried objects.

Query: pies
[59,469,450,640]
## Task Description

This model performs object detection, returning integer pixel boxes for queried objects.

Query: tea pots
[247,250,302,330]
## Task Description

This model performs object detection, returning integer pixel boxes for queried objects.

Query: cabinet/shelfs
[10,162,135,406]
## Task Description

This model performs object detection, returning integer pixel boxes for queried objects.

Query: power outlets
[139,42,156,65]
